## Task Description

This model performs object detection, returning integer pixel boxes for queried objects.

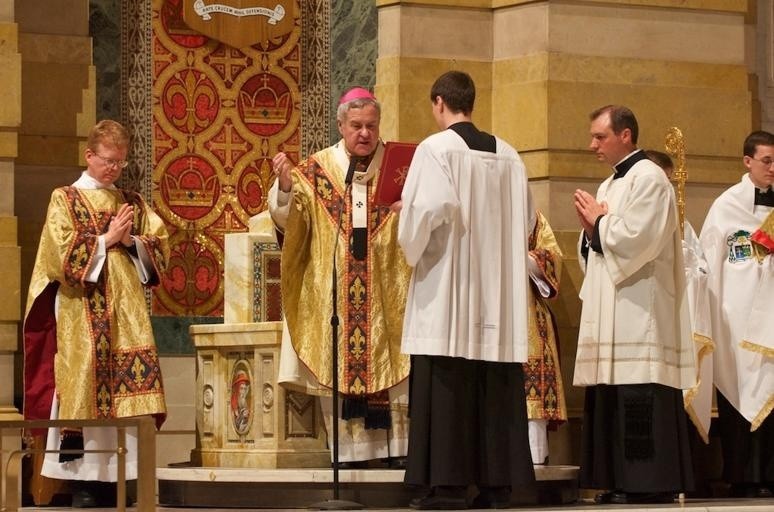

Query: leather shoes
[594,487,675,505]
[71,484,99,507]
[102,490,133,508]
[756,485,773,498]
[331,459,368,470]
[387,456,408,468]
[473,489,513,509]
[409,486,468,511]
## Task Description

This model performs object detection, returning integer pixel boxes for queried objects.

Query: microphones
[343,156,358,184]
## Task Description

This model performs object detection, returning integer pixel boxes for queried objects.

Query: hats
[338,85,376,105]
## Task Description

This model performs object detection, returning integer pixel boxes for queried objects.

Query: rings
[275,166,281,175]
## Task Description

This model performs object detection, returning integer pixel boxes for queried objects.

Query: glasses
[94,152,129,169]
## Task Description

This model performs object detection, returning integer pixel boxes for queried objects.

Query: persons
[391,71,539,511]
[698,129,774,496]
[575,102,697,505]
[267,86,419,471]
[230,370,252,432]
[20,119,171,508]
[527,207,569,465]
[644,150,726,498]
